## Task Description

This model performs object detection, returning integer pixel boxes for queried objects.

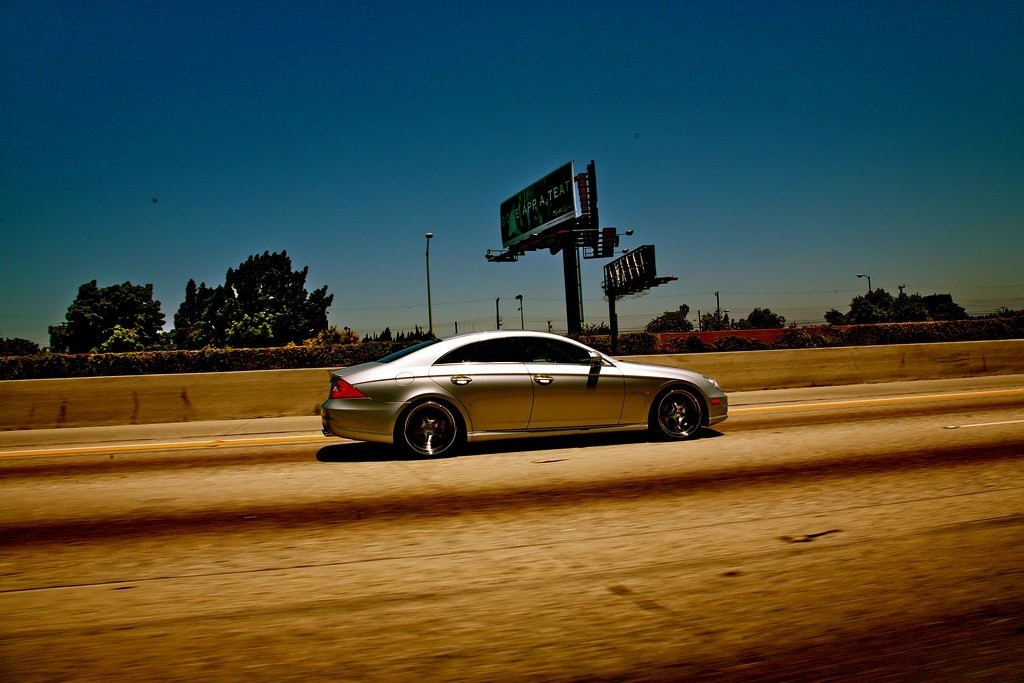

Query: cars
[318,329,729,459]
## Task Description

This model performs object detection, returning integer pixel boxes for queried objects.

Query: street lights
[425,231,434,335]
[516,294,525,329]
[856,272,872,297]
